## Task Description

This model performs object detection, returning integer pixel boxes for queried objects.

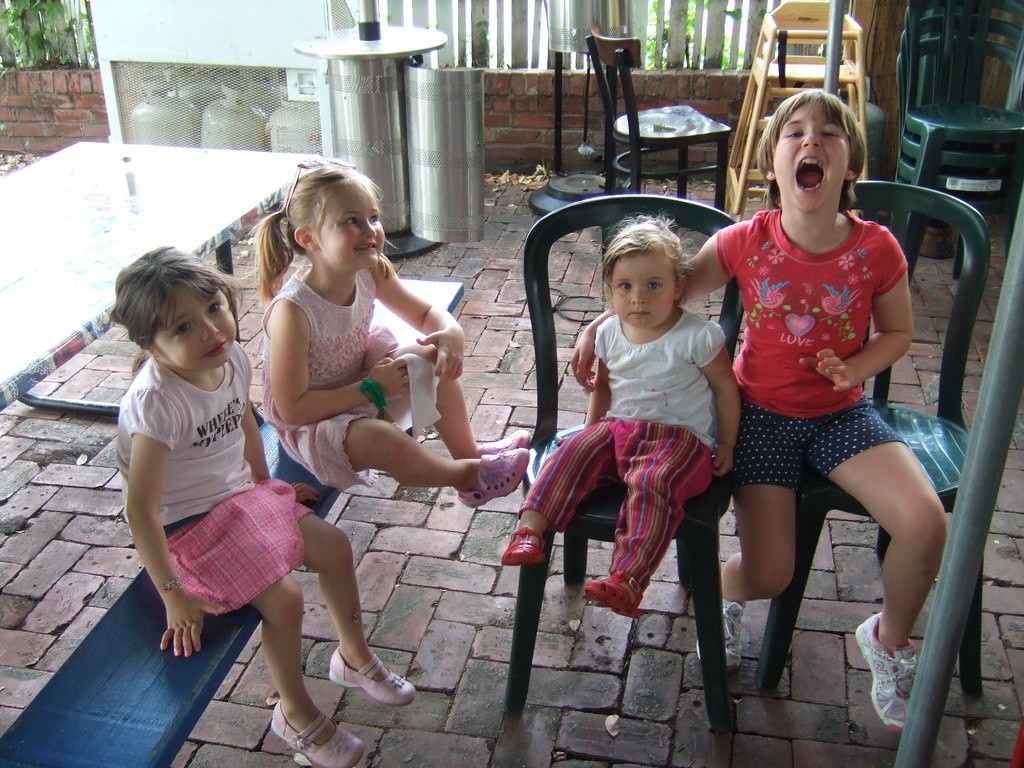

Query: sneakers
[696,598,746,671]
[856,612,919,734]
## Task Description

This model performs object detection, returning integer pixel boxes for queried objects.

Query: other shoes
[271,699,365,768]
[328,648,416,707]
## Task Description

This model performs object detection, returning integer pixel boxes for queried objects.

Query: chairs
[586,22,731,214]
[754,179,983,699]
[890,0,1024,286]
[503,195,744,733]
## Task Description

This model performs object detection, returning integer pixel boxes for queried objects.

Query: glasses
[285,154,358,225]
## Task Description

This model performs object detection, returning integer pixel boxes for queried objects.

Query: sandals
[456,448,530,508]
[480,429,531,456]
[501,527,547,566]
[585,569,644,617]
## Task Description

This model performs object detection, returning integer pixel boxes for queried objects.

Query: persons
[109,243,418,768]
[264,158,533,508]
[500,211,743,619]
[569,90,951,729]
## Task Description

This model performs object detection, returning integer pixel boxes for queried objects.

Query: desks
[1,142,326,427]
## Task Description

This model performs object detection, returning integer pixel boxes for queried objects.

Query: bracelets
[158,576,184,592]
[717,441,735,451]
[360,377,388,421]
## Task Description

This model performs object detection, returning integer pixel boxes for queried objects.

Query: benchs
[1,279,465,768]
